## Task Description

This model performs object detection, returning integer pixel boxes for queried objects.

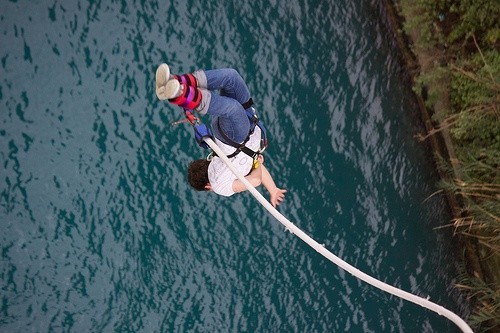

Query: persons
[155,63,287,209]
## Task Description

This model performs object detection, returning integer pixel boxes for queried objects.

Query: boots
[154,61,208,93]
[156,78,212,118]
